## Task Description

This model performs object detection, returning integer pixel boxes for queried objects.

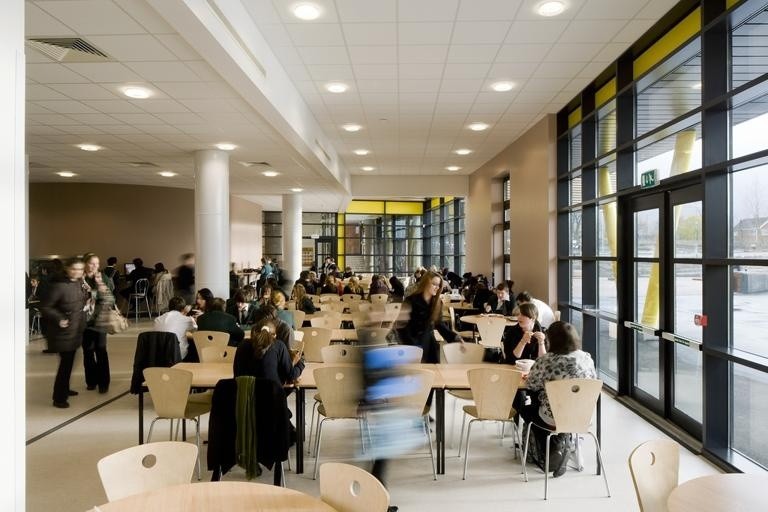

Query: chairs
[319,463,390,512]
[97,440,199,501]
[628,439,682,512]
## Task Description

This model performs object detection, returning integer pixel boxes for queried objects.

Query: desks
[86,481,338,512]
[667,472,768,512]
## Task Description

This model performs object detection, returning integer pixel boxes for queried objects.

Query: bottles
[337,281,342,296]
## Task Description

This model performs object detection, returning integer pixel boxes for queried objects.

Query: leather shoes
[543,450,562,472]
[69,391,78,395]
[54,400,69,408]
[553,448,570,477]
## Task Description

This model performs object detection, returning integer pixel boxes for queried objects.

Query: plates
[488,314,496,317]
[508,316,518,321]
[496,314,504,318]
[480,314,489,317]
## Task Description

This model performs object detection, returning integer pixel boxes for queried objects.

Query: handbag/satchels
[95,304,129,334]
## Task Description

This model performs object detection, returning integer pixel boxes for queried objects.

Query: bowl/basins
[516,359,535,371]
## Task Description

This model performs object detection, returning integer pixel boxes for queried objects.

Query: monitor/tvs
[124,263,136,274]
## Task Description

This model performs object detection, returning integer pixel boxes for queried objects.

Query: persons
[26,251,560,426]
[520,320,597,478]
